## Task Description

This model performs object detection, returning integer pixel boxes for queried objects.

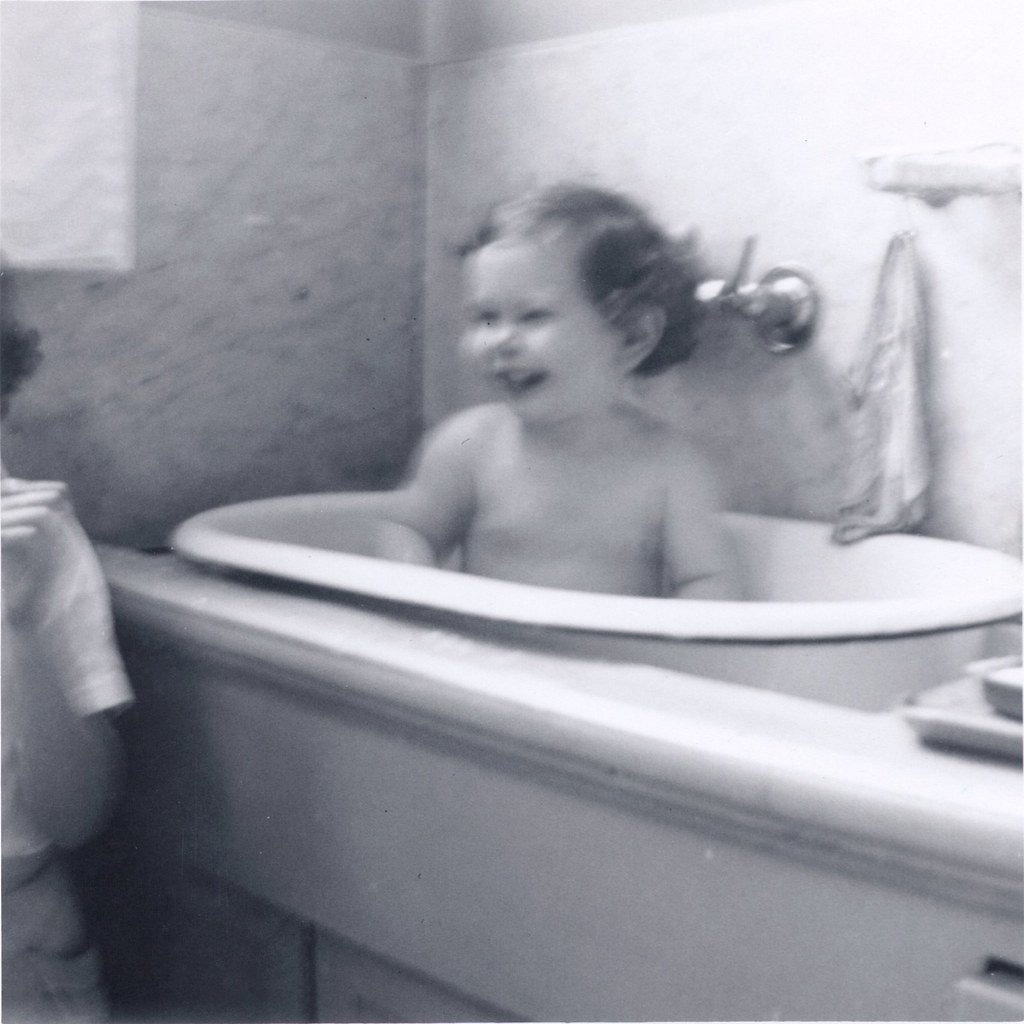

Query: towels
[822,229,937,548]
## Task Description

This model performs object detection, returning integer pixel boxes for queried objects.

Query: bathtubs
[158,481,1024,725]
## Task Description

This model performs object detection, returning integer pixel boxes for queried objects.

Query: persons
[1,249,136,1022]
[370,179,741,604]
